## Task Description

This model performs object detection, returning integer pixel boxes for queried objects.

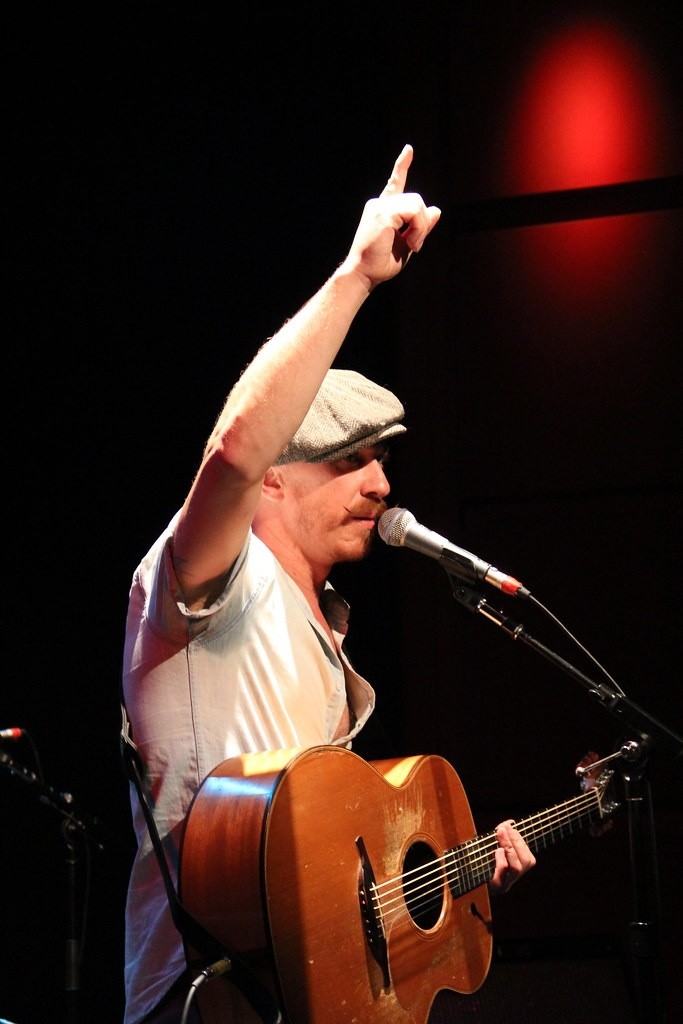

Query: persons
[123,144,537,1024]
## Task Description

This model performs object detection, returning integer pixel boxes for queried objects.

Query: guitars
[178,747,625,1023]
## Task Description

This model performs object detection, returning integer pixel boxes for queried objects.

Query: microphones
[0,728,26,741]
[378,507,533,599]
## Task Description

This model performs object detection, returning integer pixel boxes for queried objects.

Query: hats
[272,369,407,466]
